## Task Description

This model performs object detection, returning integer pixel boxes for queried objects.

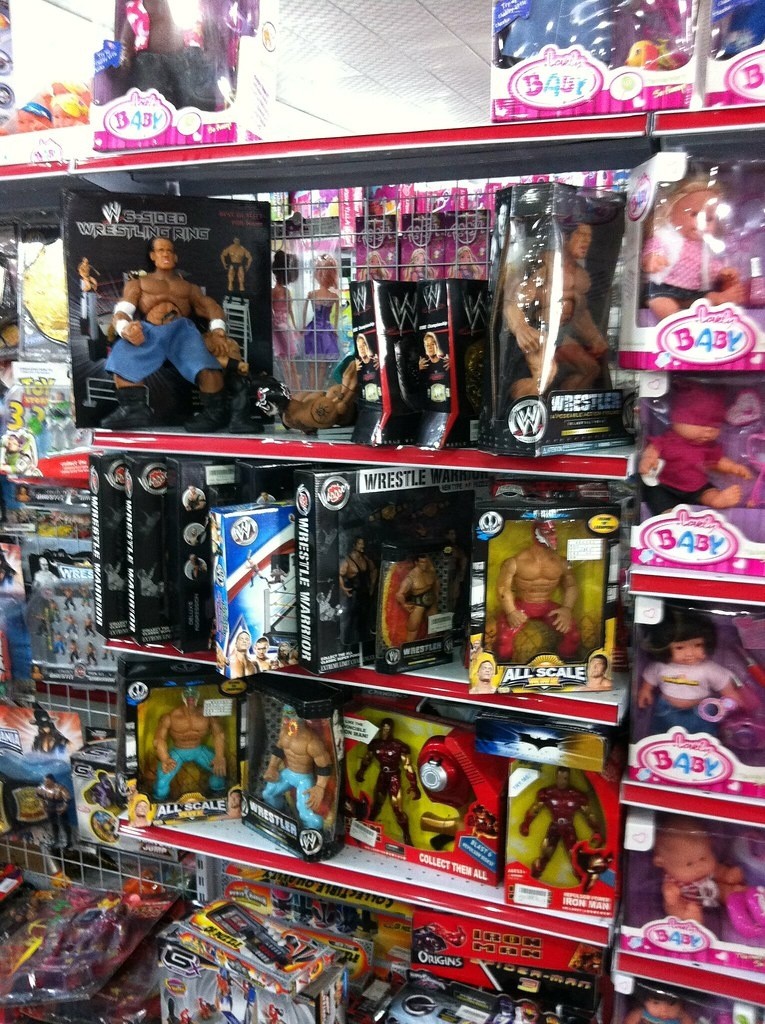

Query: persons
[1,78,91,135]
[1,211,614,888]
[624,177,757,1024]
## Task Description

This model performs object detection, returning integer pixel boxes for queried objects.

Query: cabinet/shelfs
[76,114,622,1024]
[622,100,765,1024]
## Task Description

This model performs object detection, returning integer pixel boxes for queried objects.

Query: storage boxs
[0,0,765,1024]
